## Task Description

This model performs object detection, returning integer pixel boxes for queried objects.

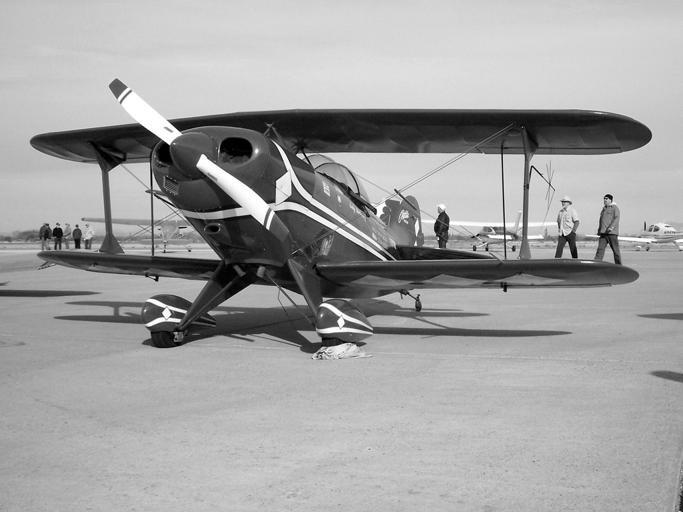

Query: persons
[555,196,580,258]
[39,221,95,251]
[594,194,622,264]
[434,203,450,248]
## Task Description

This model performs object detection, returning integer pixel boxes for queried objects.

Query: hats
[438,203,447,212]
[561,197,572,204]
[604,194,613,200]
